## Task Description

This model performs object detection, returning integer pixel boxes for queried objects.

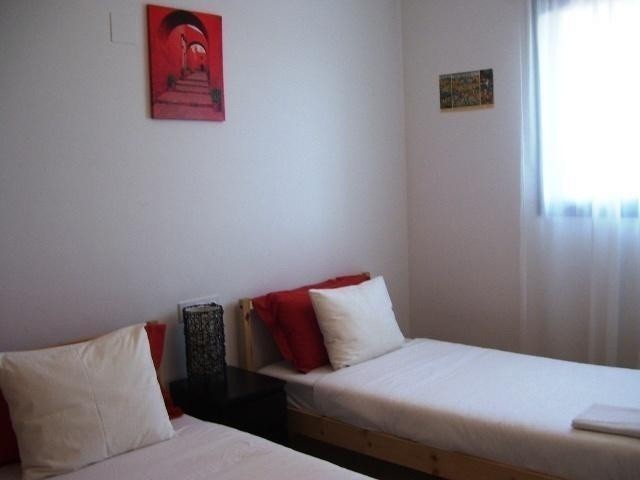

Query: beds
[232,266,640,480]
[0,316,384,479]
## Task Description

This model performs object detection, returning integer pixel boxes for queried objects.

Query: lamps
[181,300,229,393]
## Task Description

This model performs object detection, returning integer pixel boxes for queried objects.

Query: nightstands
[164,362,293,449]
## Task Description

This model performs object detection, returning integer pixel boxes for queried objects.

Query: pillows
[144,319,184,421]
[305,275,408,374]
[0,317,182,478]
[248,271,381,372]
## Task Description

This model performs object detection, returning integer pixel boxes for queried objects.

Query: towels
[567,404,640,440]
